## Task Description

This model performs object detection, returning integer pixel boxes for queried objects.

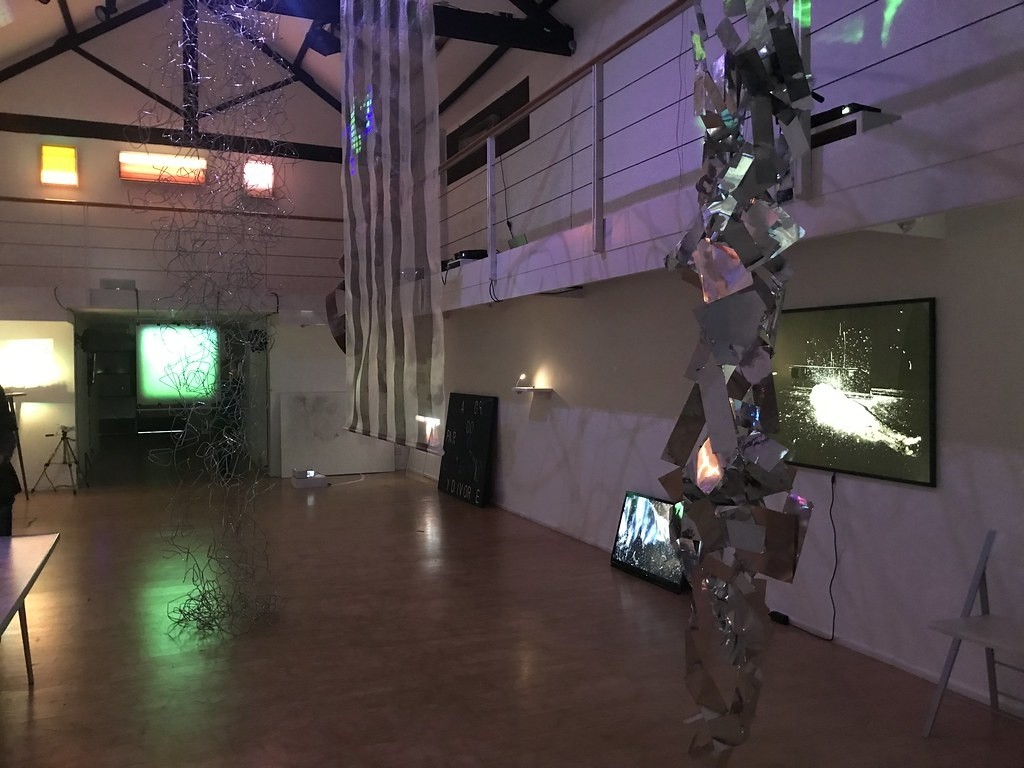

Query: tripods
[32,428,90,495]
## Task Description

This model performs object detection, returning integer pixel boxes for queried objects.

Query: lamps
[304,21,340,57]
[95,0,117,22]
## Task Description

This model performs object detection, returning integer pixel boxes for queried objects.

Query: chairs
[919,529,1024,738]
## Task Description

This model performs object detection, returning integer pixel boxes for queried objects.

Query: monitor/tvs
[741,296,939,486]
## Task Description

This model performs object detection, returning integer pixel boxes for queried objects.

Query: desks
[0,532,60,685]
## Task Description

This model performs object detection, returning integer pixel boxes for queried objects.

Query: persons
[0,386,22,536]
